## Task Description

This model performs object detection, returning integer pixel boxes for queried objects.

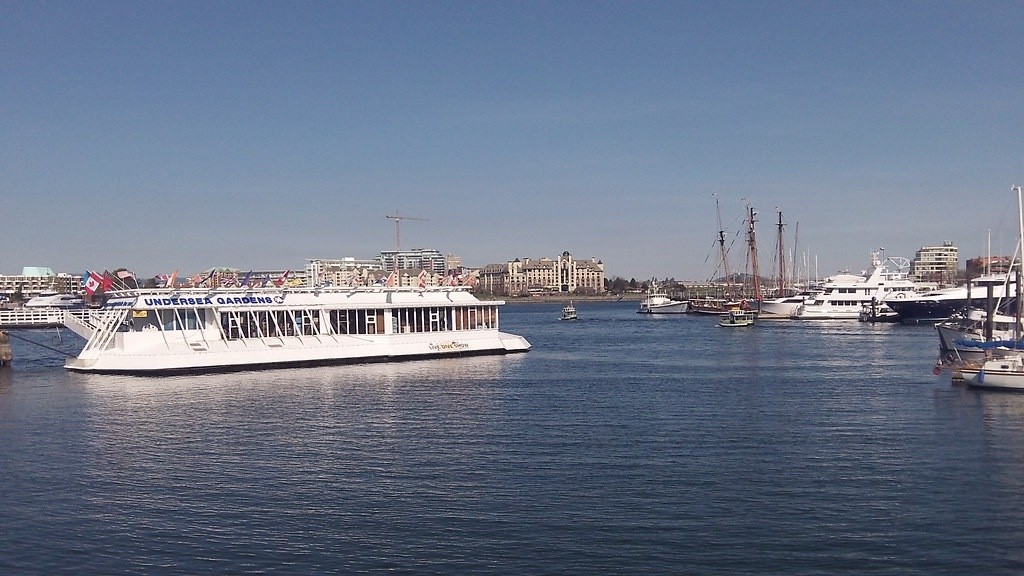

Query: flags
[83,270,132,295]
[155,269,303,288]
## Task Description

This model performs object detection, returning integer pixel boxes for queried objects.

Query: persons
[353,266,368,281]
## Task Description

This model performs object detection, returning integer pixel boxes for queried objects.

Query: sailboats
[688,192,805,322]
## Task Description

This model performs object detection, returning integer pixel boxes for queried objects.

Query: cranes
[385,209,429,251]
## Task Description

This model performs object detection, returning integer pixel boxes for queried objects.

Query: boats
[62,286,531,376]
[738,249,938,320]
[636,282,690,313]
[718,309,757,327]
[934,185,1024,388]
[882,228,1024,321]
[561,301,577,321]
